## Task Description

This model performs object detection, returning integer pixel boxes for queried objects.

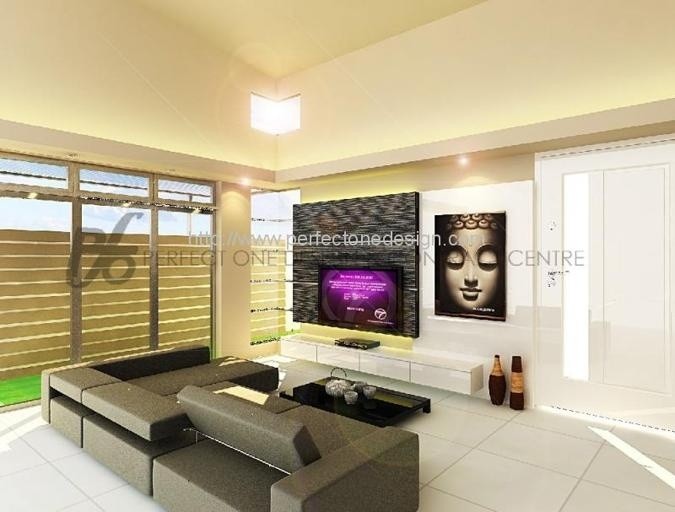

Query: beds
[292,373,431,426]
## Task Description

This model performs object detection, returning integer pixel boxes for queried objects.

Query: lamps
[246,90,304,139]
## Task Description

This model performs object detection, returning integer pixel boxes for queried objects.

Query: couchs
[39,342,421,511]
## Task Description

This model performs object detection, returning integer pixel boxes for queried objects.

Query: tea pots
[325,367,357,398]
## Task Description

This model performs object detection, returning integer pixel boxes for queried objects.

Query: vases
[488,355,506,405]
[511,356,525,409]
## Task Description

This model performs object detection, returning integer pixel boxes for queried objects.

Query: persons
[434,213,503,318]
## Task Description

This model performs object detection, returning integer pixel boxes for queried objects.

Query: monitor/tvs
[318,265,404,334]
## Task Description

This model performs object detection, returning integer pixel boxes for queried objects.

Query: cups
[343,391,358,406]
[354,380,376,399]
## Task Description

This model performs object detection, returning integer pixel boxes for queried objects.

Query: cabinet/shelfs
[280,332,485,396]
